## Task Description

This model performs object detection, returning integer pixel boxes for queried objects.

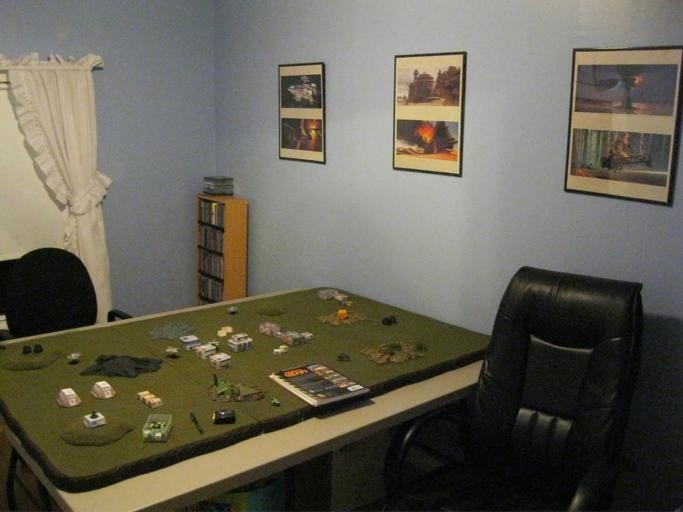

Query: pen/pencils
[189,412,203,433]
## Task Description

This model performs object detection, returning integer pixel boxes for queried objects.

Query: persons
[614,131,635,160]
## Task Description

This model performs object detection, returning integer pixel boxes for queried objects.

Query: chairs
[0,248,134,512]
[385,266,644,512]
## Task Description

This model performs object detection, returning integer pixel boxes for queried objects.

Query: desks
[0,288,490,512]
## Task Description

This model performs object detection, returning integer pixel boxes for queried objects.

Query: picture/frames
[392,52,467,177]
[565,45,682,207]
[279,63,326,164]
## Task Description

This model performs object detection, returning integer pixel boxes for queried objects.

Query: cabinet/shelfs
[195,195,249,305]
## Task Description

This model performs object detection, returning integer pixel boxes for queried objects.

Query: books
[269,361,372,408]
[203,173,234,196]
[197,198,223,307]
